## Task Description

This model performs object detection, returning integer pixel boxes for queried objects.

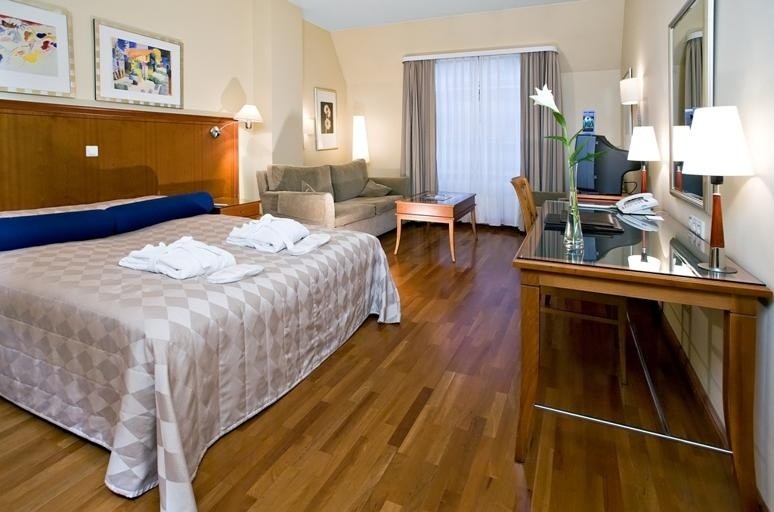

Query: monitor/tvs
[574,134,641,196]
[583,221,641,262]
[683,108,703,197]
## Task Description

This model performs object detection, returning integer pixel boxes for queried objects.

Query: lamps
[210,102,265,140]
[668,121,689,181]
[680,106,758,276]
[616,64,645,110]
[628,123,664,195]
[351,115,370,164]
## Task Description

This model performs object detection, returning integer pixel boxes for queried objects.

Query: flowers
[527,87,632,250]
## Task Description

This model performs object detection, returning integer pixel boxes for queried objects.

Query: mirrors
[667,0,717,214]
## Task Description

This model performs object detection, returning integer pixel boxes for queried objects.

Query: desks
[518,195,771,508]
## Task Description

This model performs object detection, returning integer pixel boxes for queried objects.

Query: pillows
[358,177,394,196]
[1,190,113,249]
[106,188,219,238]
[300,179,315,192]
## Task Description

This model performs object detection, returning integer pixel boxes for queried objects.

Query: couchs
[254,158,415,244]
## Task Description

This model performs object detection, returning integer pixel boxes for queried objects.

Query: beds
[0,97,405,510]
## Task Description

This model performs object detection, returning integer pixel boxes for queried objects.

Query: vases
[562,184,587,247]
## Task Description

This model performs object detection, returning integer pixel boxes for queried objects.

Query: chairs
[509,175,630,378]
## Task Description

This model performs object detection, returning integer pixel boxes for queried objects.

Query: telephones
[615,193,659,214]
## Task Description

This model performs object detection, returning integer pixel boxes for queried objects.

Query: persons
[583,111,594,129]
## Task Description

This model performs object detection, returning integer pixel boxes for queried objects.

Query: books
[420,193,454,201]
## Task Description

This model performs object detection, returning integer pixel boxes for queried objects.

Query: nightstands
[212,195,263,220]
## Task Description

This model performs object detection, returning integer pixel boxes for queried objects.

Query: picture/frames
[0,1,77,100]
[313,86,337,151]
[92,18,185,110]
